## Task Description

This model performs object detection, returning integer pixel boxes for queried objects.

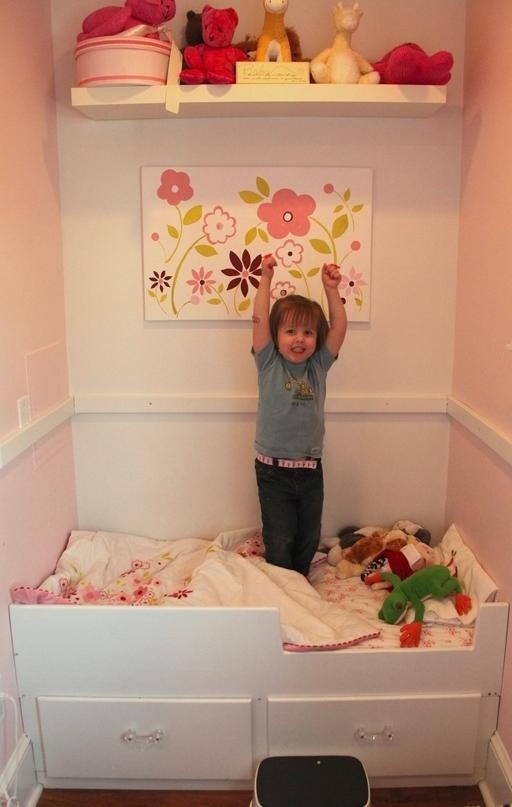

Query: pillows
[399,524,499,628]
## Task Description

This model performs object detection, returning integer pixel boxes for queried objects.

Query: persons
[249,253,349,577]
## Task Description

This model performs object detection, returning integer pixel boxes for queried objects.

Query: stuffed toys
[309,1,381,84]
[178,4,249,85]
[254,0,293,62]
[76,0,176,42]
[180,11,203,70]
[373,43,453,85]
[317,520,472,648]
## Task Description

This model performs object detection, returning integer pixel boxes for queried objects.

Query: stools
[249,754,373,807]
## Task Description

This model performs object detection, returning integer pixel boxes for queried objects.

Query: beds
[8,530,511,790]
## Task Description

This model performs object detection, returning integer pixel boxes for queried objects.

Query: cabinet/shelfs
[33,692,483,781]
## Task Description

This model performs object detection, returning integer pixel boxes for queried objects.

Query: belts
[256,452,318,469]
